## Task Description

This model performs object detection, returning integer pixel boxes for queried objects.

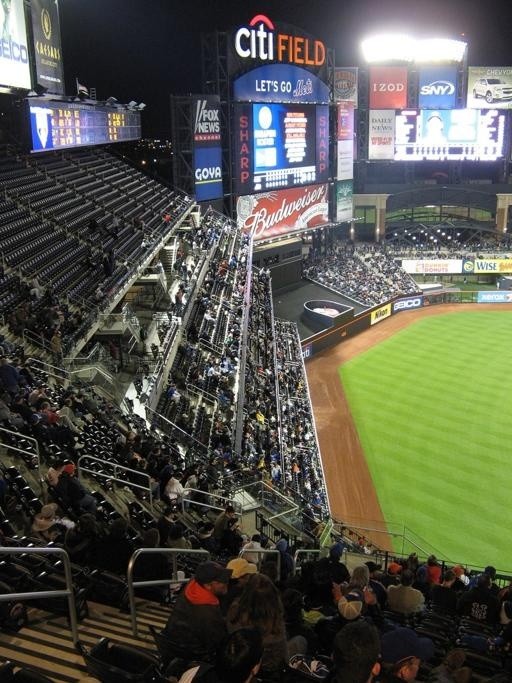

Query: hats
[0,349,238,533]
[225,558,257,580]
[337,588,363,620]
[196,560,233,584]
[364,560,428,581]
[381,627,435,663]
[277,538,346,580]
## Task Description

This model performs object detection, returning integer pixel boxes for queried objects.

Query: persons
[1,192,333,522]
[301,231,512,308]
[422,112,447,143]
[0,519,509,683]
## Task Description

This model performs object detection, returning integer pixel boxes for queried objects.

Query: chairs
[326,212,511,306]
[0,150,327,552]
[1,538,512,675]
